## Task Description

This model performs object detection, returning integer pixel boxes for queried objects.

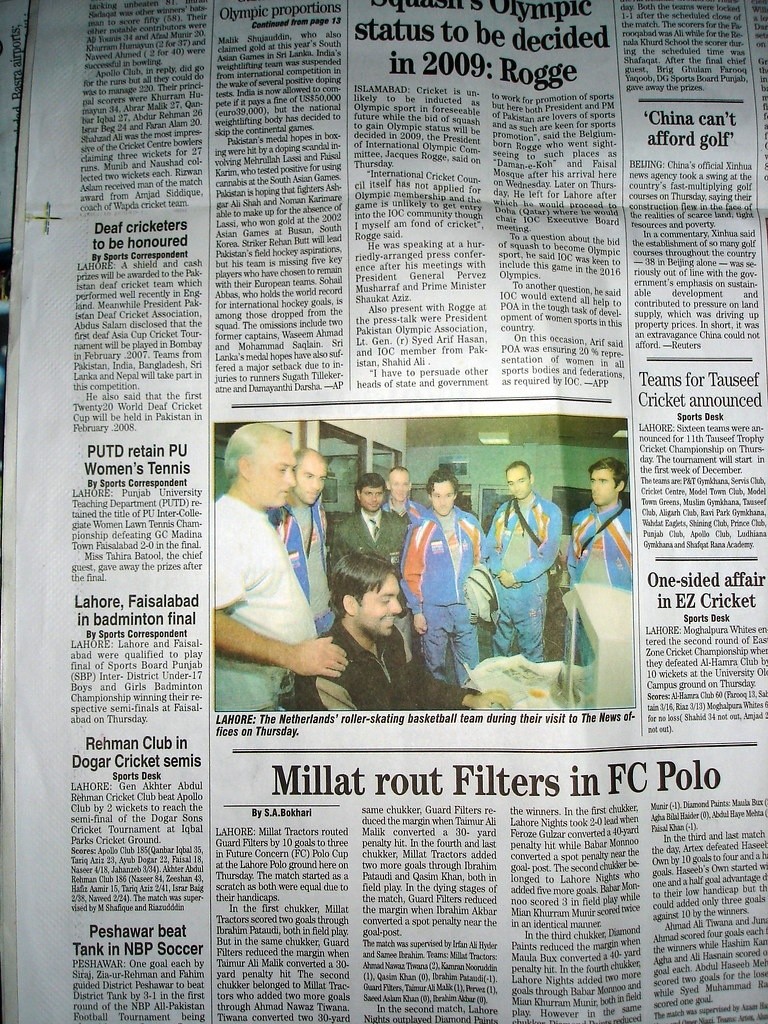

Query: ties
[369,518,381,542]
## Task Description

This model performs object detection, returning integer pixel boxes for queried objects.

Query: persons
[215,424,350,712]
[265,448,631,711]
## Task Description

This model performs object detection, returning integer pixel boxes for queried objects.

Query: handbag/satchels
[547,555,563,589]
